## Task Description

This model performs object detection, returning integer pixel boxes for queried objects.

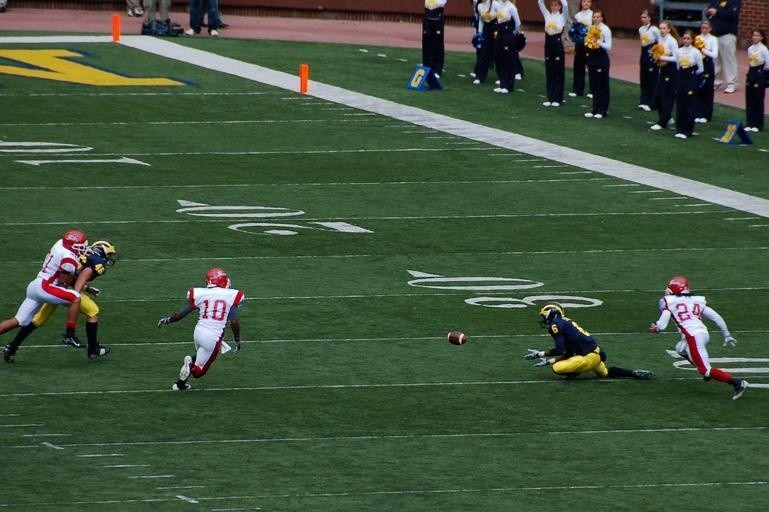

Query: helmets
[665,277,690,296]
[205,268,232,289]
[540,303,565,324]
[84,240,116,268]
[64,229,88,257]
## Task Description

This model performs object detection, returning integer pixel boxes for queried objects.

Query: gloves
[158,316,172,327]
[524,349,545,359]
[84,285,100,298]
[233,341,241,353]
[534,358,555,366]
[723,336,737,347]
[649,324,659,333]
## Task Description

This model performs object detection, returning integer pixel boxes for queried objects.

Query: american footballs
[447,332,466,344]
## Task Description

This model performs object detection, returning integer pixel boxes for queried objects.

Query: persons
[523,303,655,381]
[0,228,88,348]
[469,0,525,94]
[649,276,749,401]
[126,0,230,38]
[157,265,246,391]
[538,0,613,120]
[4,240,117,362]
[637,0,741,139]
[422,0,447,79]
[742,27,769,132]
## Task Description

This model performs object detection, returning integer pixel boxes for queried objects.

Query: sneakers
[62,333,86,348]
[89,345,111,359]
[632,369,657,379]
[127,8,229,38]
[731,379,747,400]
[172,383,192,391]
[179,356,193,381]
[3,343,16,363]
[638,86,759,140]
[470,72,522,93]
[542,92,603,120]
